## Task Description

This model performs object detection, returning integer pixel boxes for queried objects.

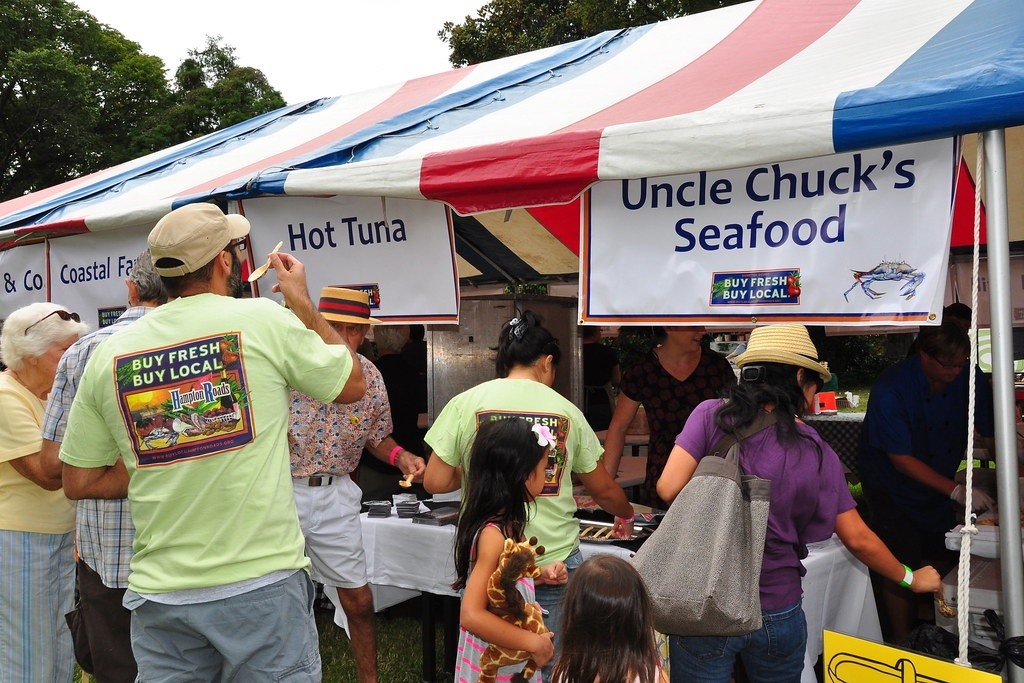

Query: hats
[318,287,382,325]
[148,203,250,276]
[734,322,831,383]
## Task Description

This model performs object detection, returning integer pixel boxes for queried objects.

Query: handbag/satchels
[633,397,772,638]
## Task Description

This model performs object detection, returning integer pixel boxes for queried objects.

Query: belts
[290,475,336,486]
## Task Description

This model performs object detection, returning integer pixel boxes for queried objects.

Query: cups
[396,501,420,518]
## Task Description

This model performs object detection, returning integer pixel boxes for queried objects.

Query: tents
[0,0,1024,683]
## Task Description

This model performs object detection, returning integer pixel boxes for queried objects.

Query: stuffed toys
[478,536,553,683]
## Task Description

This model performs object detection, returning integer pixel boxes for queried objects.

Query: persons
[852,302,996,640]
[39,250,177,683]
[584,325,621,431]
[603,326,738,509]
[0,302,89,683]
[450,419,568,683]
[290,286,427,683]
[423,309,635,683]
[656,324,941,683]
[57,202,365,683]
[548,554,669,683]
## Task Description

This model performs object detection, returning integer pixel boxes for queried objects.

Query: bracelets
[620,512,635,524]
[898,564,913,587]
[389,446,403,464]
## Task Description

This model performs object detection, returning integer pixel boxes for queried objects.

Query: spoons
[399,474,414,487]
[248,241,283,282]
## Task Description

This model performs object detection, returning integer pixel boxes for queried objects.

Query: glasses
[932,356,966,370]
[224,237,246,251]
[25,311,80,335]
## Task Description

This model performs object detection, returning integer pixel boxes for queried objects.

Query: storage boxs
[934,553,1004,650]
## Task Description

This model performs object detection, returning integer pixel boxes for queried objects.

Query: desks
[432,455,648,503]
[322,499,883,683]
[595,430,650,457]
